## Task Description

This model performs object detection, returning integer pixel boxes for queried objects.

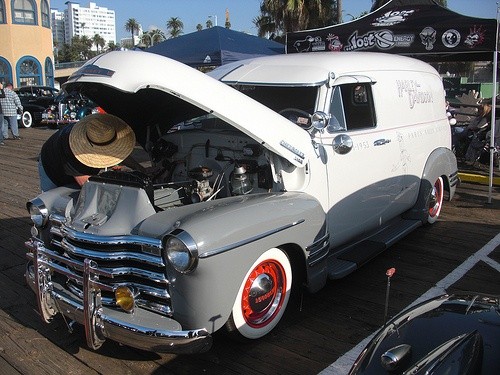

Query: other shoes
[4,138,9,140]
[0,142,7,145]
[14,136,22,140]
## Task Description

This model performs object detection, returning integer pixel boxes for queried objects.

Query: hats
[69,113,135,168]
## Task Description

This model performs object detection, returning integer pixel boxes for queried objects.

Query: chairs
[341,83,373,130]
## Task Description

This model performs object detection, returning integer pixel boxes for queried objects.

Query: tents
[285,1,500,204]
[142,25,286,68]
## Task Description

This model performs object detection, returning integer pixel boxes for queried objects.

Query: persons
[0,82,23,145]
[37,113,136,193]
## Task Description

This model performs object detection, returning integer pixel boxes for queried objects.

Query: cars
[42,85,94,128]
[23,48,461,355]
[15,86,60,128]
